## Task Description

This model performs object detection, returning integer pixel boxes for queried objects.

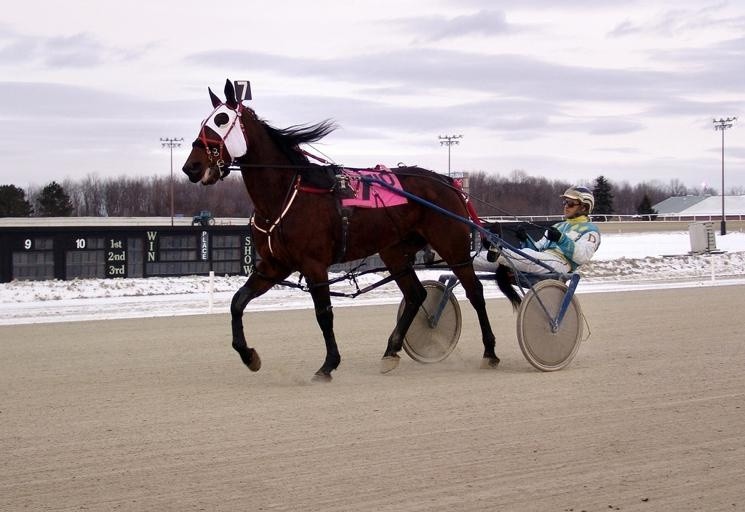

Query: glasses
[563,200,580,208]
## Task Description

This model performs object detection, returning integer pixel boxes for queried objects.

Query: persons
[423,185,601,273]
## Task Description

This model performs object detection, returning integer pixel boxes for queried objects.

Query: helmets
[559,184,595,213]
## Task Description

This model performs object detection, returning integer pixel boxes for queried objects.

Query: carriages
[180,79,585,383]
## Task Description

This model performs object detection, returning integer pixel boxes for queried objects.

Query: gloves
[547,226,561,241]
[516,225,527,242]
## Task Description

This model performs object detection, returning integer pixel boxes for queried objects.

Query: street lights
[160,136,184,228]
[711,115,737,236]
[438,133,463,177]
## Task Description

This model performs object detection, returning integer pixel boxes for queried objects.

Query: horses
[181,77,523,384]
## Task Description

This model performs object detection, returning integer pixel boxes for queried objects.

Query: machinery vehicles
[191,209,215,226]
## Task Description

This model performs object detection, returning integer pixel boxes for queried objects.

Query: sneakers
[424,246,435,267]
[486,222,502,263]
